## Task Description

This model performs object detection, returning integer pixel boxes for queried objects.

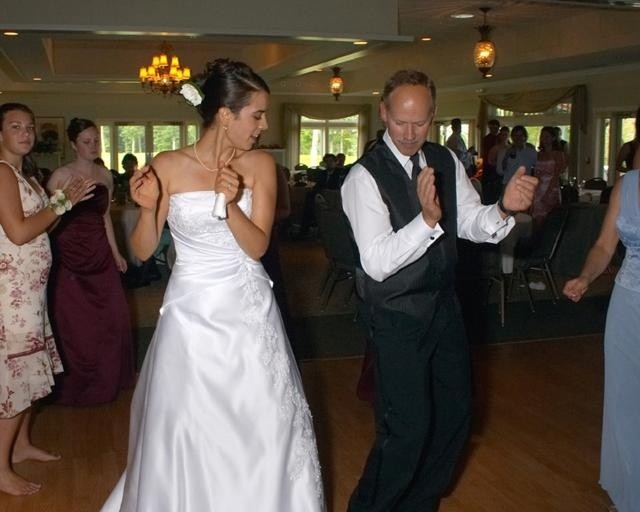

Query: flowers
[177,80,206,107]
[47,189,73,216]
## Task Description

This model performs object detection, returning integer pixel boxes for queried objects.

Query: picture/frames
[32,115,66,188]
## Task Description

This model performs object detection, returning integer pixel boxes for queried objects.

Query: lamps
[138,41,192,98]
[329,63,344,100]
[471,6,496,78]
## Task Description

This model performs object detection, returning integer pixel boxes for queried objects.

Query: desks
[109,198,143,268]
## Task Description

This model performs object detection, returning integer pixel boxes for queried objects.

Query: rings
[227,182,232,190]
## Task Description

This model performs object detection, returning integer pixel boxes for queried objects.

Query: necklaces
[193,142,236,173]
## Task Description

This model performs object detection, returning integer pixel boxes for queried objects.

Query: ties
[411,150,459,279]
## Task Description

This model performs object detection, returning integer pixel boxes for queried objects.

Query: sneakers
[521,282,545,290]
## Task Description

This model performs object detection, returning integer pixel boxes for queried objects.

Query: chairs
[315,189,356,302]
[316,202,364,323]
[479,253,537,329]
[560,177,613,205]
[509,207,570,305]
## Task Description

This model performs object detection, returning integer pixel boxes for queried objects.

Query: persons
[335,151,346,170]
[553,127,569,169]
[616,107,640,173]
[488,126,511,204]
[481,119,511,166]
[98,57,329,511]
[115,154,139,209]
[0,103,96,496]
[293,154,341,241]
[562,151,640,511]
[496,125,538,195]
[46,117,128,404]
[339,70,539,511]
[443,118,478,172]
[530,126,563,225]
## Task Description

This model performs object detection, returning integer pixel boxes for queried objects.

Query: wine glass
[577,179,587,192]
[569,176,576,188]
